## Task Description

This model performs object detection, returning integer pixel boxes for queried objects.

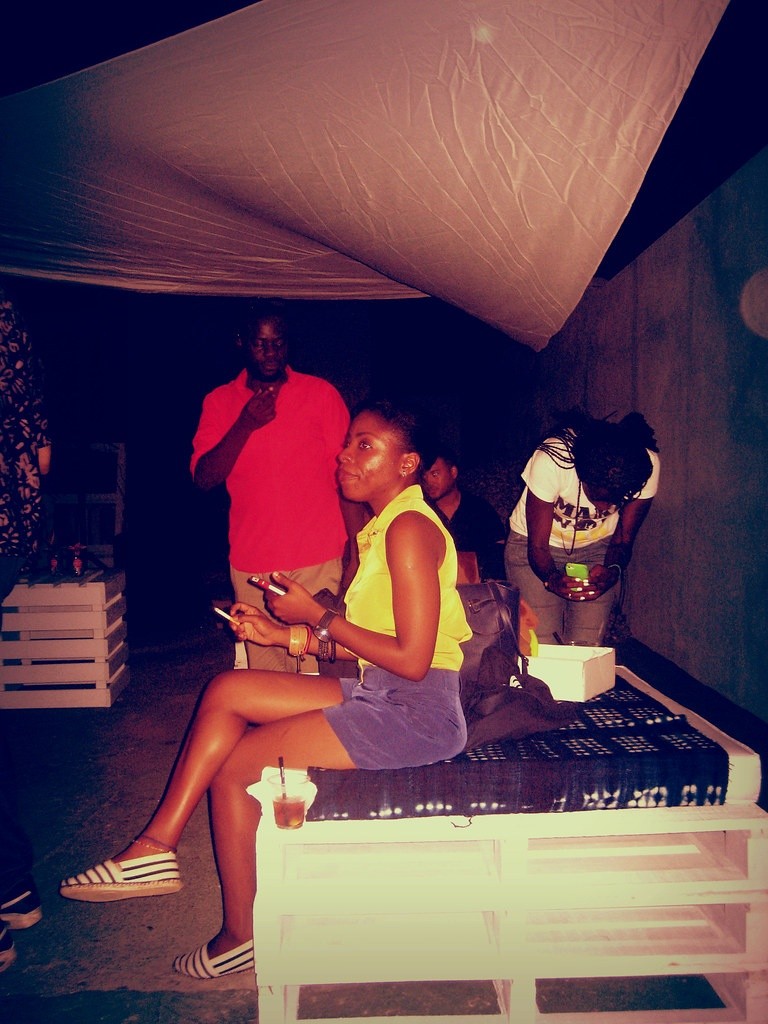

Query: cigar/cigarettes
[214,606,240,627]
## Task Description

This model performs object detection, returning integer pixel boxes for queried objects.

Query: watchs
[313,608,338,643]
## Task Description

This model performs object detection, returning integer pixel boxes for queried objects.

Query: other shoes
[172,941,255,979]
[0,920,15,972]
[0,891,41,929]
[60,850,181,902]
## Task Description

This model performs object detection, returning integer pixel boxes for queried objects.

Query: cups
[267,773,311,830]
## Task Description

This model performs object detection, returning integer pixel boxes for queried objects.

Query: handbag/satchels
[455,579,521,681]
[461,647,579,750]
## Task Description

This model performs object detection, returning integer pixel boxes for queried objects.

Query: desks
[0,568,129,710]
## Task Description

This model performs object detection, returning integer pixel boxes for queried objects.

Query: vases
[73,556,83,575]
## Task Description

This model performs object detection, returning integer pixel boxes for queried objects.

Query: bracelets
[609,564,622,580]
[322,642,329,662]
[330,639,337,663]
[288,624,311,674]
[317,641,322,661]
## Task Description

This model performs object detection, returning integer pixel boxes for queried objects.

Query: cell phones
[250,575,287,596]
[566,563,589,592]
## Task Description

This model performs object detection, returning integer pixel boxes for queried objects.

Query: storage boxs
[517,644,614,702]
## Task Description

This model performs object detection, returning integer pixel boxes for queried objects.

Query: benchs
[251,668,768,1024]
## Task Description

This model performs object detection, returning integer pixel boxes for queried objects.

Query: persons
[423,455,507,583]
[0,762,42,971]
[59,394,478,980]
[189,304,365,674]
[504,408,661,649]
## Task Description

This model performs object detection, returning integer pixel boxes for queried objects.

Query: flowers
[68,543,87,550]
